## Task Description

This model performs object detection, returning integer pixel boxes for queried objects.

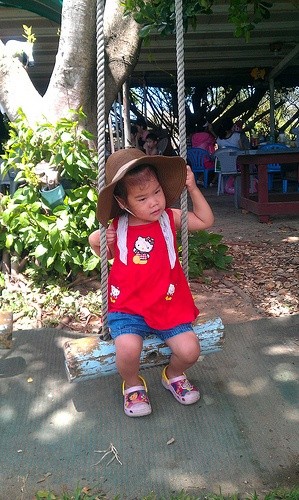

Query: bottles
[279,132,285,144]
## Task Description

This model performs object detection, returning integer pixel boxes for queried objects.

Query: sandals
[122,375,152,417]
[161,365,200,405]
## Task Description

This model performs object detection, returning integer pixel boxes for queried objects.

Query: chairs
[187,144,291,195]
[157,138,168,155]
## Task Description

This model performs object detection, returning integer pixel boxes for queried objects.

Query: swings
[63,0,225,384]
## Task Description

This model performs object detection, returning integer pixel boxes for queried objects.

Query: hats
[97,148,187,229]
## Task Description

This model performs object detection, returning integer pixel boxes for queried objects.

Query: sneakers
[210,182,217,187]
[224,184,235,193]
[197,180,204,186]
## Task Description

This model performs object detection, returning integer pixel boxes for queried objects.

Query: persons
[89,148,214,417]
[131,116,249,187]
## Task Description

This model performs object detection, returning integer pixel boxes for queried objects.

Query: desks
[236,153,299,223]
